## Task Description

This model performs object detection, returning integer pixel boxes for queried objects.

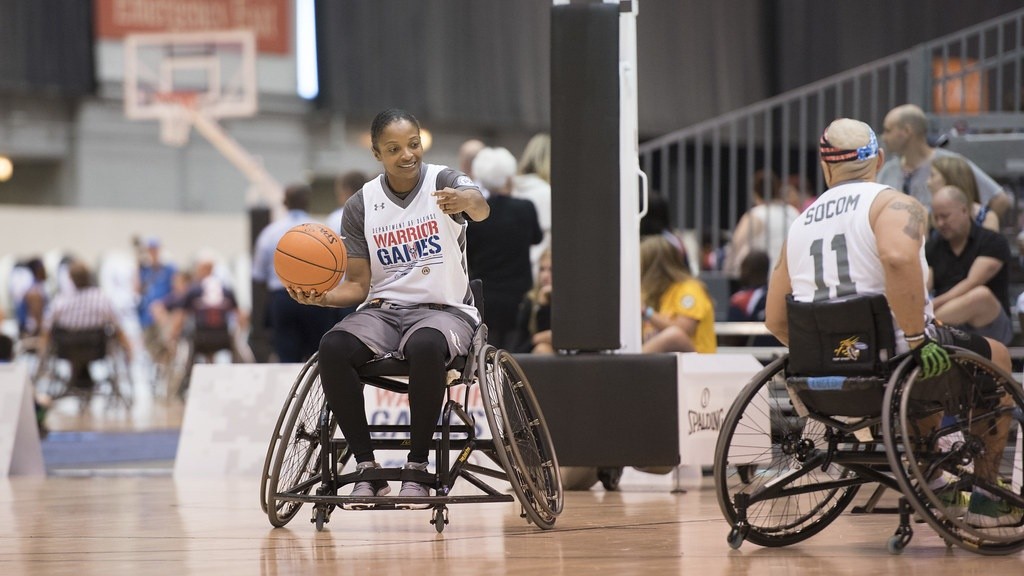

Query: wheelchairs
[714,293,1024,558]
[32,323,136,412]
[146,322,256,411]
[260,279,565,530]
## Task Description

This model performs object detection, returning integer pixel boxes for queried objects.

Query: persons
[637,232,717,354]
[0,132,555,414]
[764,119,1024,527]
[283,110,490,510]
[718,108,1017,347]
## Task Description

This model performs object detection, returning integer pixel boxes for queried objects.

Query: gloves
[904,335,951,382]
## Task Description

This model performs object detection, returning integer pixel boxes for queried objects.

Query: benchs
[707,319,797,413]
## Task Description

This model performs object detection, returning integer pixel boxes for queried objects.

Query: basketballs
[273,223,347,297]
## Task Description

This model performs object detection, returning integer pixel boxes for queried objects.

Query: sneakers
[963,480,1024,543]
[396,461,431,509]
[343,468,389,511]
[912,481,971,523]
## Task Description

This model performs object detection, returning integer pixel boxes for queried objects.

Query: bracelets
[645,307,654,320]
[903,332,924,342]
